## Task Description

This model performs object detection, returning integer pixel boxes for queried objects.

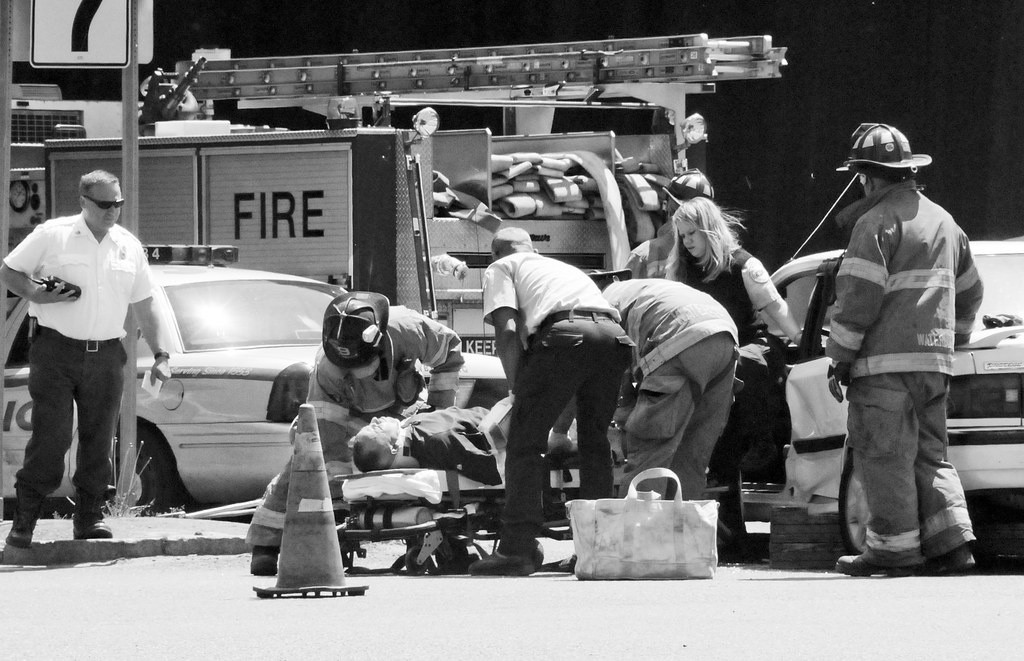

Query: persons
[602,167,803,564]
[246,291,465,576]
[352,406,625,485]
[468,227,633,576]
[827,122,984,577]
[0,170,171,548]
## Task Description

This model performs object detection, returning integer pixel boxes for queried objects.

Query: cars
[1,240,354,518]
[736,237,1024,562]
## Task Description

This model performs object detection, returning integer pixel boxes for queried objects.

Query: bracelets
[154,352,169,359]
[793,331,802,344]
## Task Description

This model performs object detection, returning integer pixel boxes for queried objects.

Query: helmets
[322,291,390,368]
[662,168,715,206]
[843,123,933,173]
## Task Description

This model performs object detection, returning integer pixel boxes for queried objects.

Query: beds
[335,456,628,575]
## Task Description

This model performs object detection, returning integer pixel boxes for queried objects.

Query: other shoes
[250,546,279,575]
[914,543,975,576]
[543,554,577,571]
[835,555,924,577]
[467,548,535,577]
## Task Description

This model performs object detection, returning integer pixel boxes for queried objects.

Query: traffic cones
[252,402,371,600]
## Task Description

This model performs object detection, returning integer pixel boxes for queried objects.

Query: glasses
[83,194,125,209]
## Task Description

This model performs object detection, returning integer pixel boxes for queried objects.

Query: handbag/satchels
[564,466,720,580]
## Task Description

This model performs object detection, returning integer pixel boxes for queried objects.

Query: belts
[540,311,611,329]
[39,327,120,352]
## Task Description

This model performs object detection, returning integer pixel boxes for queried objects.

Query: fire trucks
[8,29,790,406]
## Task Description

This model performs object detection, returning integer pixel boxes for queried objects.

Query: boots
[6,469,45,547]
[73,492,113,540]
